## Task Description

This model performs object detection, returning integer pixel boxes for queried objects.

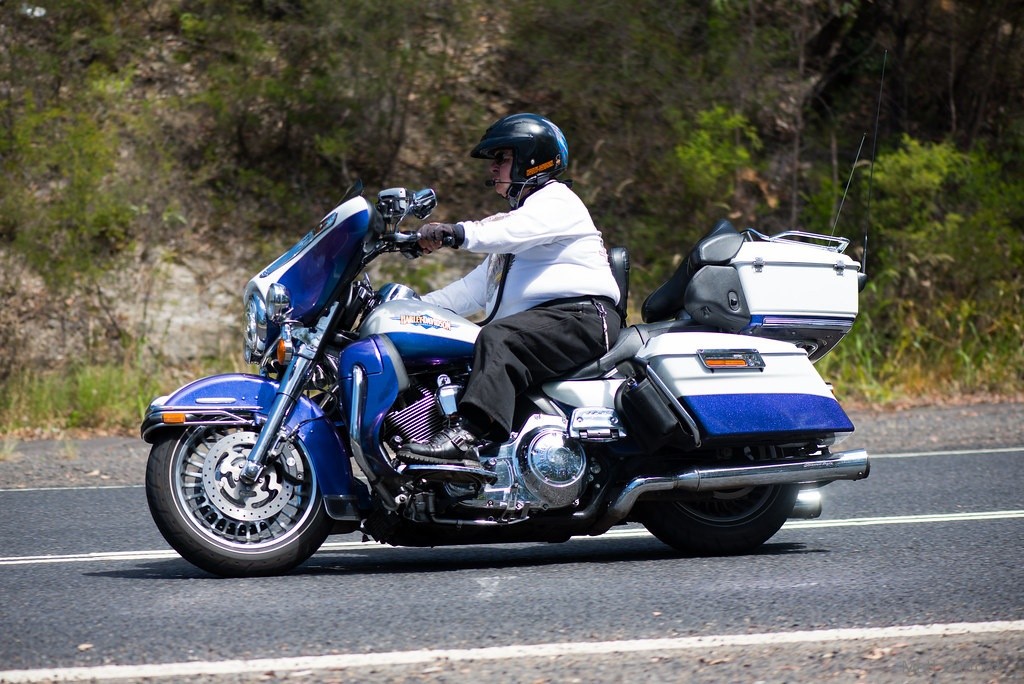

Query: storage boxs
[635,332,855,446]
[727,241,861,365]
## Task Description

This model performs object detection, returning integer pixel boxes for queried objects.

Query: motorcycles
[139,176,871,579]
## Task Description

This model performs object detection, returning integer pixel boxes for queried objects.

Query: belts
[538,297,618,310]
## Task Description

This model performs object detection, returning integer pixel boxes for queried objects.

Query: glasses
[493,150,513,166]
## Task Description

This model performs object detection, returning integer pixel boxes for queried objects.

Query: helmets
[471,113,568,183]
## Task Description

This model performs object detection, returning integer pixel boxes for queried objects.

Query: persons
[396,114,622,466]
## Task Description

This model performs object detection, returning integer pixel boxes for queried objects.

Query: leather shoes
[397,411,495,466]
